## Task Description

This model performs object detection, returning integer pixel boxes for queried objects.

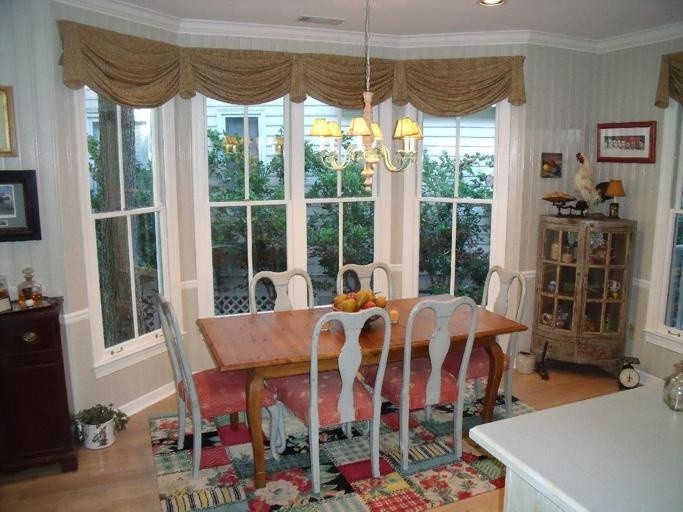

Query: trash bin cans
[516,351,536,375]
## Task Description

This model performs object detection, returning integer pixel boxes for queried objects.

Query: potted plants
[72,404,130,450]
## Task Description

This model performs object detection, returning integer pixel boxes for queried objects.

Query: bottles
[670,364,682,411]
[18,267,42,306]
[0,275,13,312]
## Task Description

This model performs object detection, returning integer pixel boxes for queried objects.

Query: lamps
[310,0,424,192]
[605,180,626,217]
[479,0,503,7]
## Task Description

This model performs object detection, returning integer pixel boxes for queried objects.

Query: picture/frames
[0,170,42,242]
[597,122,656,163]
[541,152,562,178]
[0,85,18,157]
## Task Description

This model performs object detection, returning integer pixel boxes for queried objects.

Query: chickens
[572,151,603,216]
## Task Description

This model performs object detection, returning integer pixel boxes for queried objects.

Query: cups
[317,316,330,332]
[387,309,400,326]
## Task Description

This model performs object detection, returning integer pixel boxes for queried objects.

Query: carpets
[149,380,536,511]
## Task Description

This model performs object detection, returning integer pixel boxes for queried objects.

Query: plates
[332,299,386,322]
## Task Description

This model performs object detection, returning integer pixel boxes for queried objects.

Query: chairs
[276,307,391,494]
[442,266,526,419]
[365,296,478,471]
[337,263,395,440]
[250,269,314,399]
[155,292,281,480]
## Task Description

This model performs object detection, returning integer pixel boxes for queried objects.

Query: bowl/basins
[664,371,683,412]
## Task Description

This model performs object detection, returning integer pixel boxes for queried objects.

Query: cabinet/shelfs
[0,297,78,472]
[469,380,683,512]
[531,215,637,380]
